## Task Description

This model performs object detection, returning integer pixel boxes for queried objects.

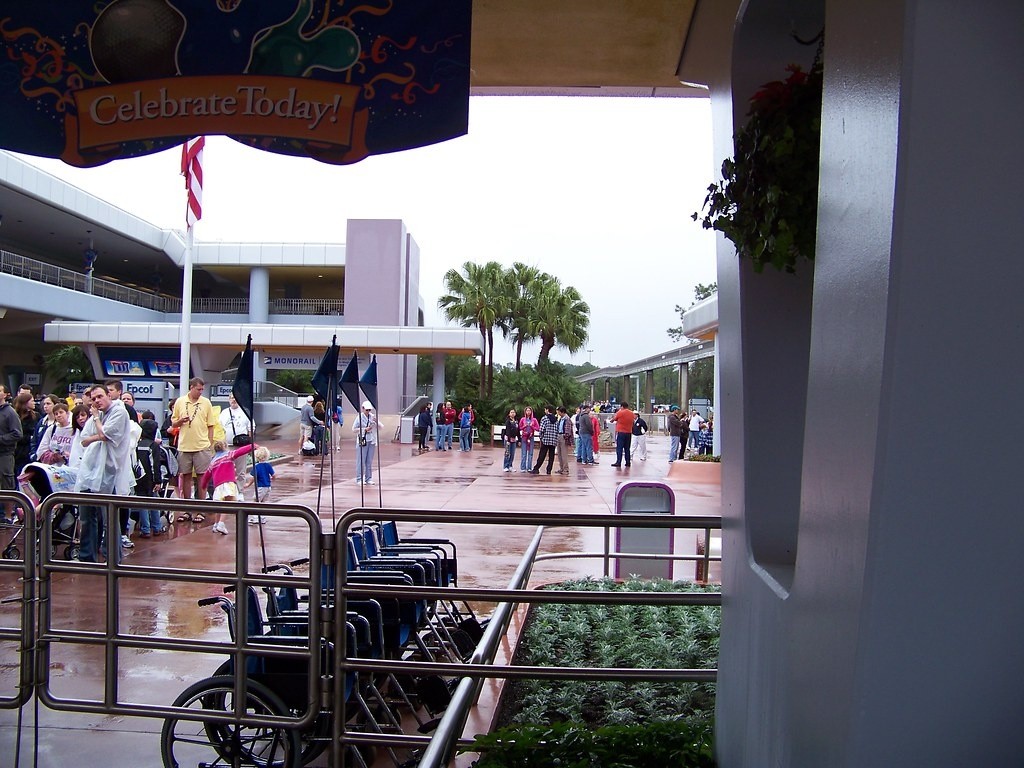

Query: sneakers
[121,534,135,548]
[212,525,228,535]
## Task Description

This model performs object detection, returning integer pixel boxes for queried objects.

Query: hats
[307,396,314,402]
[580,404,585,408]
[671,406,681,411]
[362,401,374,409]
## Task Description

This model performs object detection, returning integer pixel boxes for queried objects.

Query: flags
[181,135,205,231]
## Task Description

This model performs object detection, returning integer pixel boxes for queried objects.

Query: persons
[570,405,599,465]
[502,408,521,473]
[519,406,539,472]
[419,402,474,452]
[554,406,574,475]
[243,447,275,525]
[0,379,256,564]
[299,396,343,454]
[610,402,634,467]
[589,400,616,430]
[630,410,648,461]
[353,401,383,485]
[669,406,714,464]
[530,405,558,474]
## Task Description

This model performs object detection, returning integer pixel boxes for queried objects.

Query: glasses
[364,408,371,411]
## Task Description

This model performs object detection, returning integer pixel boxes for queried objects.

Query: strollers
[3,461,86,561]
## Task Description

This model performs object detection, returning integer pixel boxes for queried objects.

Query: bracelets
[93,417,99,421]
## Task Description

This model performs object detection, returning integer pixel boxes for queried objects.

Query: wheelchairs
[162,518,491,768]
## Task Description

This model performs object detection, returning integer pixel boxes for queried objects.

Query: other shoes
[248,517,267,524]
[298,451,304,455]
[153,530,161,536]
[0,515,15,525]
[139,532,150,538]
[329,447,341,450]
[417,444,679,476]
[12,514,19,523]
[357,481,366,485]
[368,481,375,485]
[70,558,81,562]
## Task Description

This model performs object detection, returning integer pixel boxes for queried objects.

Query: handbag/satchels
[325,429,330,441]
[233,434,253,446]
[359,437,366,447]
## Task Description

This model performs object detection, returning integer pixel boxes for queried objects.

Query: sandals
[177,512,193,522]
[192,513,206,523]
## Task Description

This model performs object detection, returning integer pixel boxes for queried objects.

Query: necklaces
[186,403,198,428]
